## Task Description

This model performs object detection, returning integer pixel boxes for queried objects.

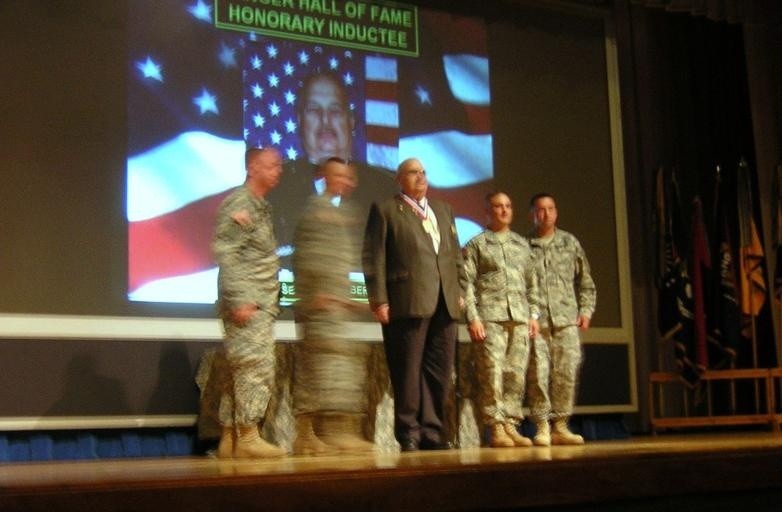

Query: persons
[211,146,287,459]
[261,72,402,249]
[360,159,469,452]
[521,192,597,447]
[280,155,375,456]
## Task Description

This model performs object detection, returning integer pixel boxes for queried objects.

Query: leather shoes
[399,433,455,451]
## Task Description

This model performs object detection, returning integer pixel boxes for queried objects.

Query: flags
[641,163,782,389]
[127,1,493,308]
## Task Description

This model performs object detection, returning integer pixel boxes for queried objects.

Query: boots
[233,422,288,459]
[505,423,533,447]
[291,414,378,458]
[489,423,515,446]
[552,422,585,445]
[532,421,552,445]
[216,426,236,458]
